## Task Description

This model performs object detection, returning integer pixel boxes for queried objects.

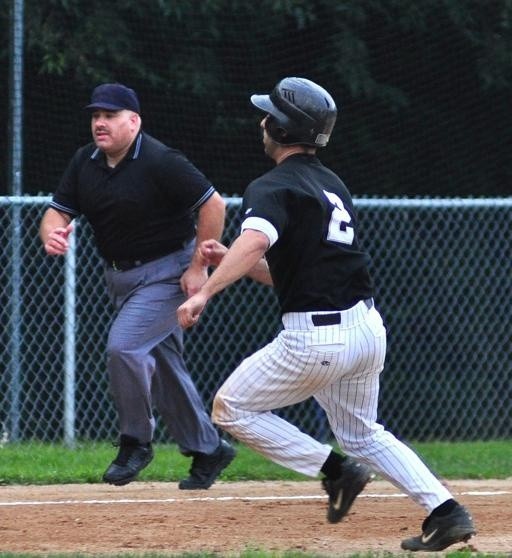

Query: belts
[311,297,373,327]
[106,240,190,271]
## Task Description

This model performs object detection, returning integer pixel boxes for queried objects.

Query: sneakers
[178,438,238,490]
[103,434,154,486]
[321,456,376,524]
[400,505,477,552]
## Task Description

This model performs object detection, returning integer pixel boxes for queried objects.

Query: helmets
[251,76,338,149]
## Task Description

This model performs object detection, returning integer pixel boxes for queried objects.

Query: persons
[173,72,480,554]
[38,81,240,491]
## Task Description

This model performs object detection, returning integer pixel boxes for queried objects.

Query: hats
[82,83,141,116]
